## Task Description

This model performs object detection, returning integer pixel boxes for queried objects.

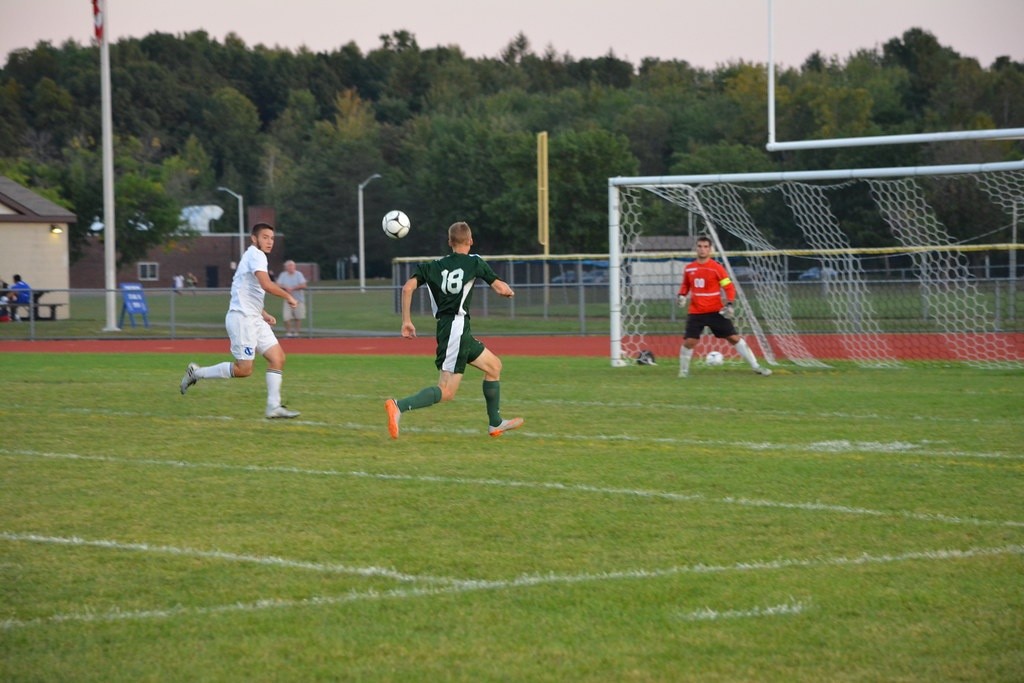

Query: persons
[172,271,198,295]
[677,237,773,378]
[179,223,300,419]
[385,221,525,439]
[276,261,307,336]
[0,274,32,322]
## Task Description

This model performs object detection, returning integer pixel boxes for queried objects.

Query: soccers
[382,210,411,239]
[706,351,723,366]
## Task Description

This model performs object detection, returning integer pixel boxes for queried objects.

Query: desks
[0,289,48,321]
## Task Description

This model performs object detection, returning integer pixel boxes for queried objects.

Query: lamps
[49,225,63,233]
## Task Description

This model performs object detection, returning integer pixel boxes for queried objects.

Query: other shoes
[753,365,772,375]
[679,370,687,377]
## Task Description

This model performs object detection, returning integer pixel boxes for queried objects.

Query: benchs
[0,302,66,321]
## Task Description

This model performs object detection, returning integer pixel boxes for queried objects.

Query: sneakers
[180,362,200,394]
[385,398,402,439]
[488,418,524,436]
[265,401,301,419]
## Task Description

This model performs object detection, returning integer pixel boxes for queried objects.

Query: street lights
[358,174,381,293]
[217,187,244,260]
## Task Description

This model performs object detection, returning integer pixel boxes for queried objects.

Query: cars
[552,268,609,284]
[799,267,838,281]
